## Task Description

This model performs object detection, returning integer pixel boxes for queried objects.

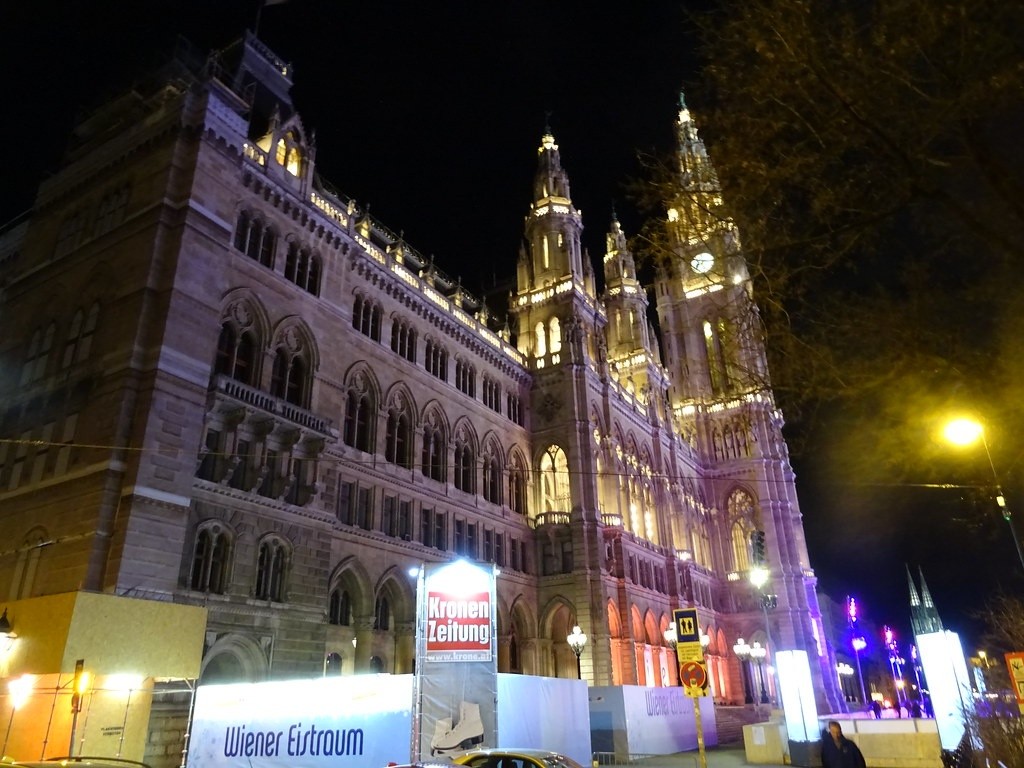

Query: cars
[435,747,586,768]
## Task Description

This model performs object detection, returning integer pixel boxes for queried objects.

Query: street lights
[749,641,770,704]
[663,621,683,686]
[566,625,587,679]
[732,637,754,705]
[940,411,1024,569]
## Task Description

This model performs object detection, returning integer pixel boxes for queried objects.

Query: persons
[821,721,866,768]
[873,697,933,719]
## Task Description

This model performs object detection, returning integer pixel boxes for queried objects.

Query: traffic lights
[751,530,764,563]
[763,594,778,609]
